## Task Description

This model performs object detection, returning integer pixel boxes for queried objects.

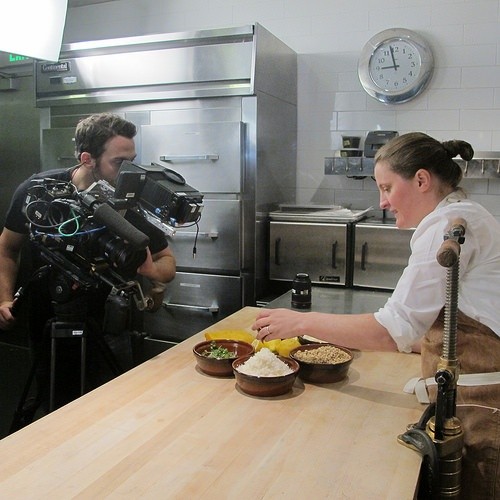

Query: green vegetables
[201,341,231,358]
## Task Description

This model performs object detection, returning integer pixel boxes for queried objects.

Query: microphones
[81,195,149,251]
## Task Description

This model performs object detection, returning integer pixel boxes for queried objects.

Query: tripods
[9,253,124,435]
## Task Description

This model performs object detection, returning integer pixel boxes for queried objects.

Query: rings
[266,327,270,334]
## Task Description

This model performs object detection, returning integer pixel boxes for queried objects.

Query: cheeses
[205,328,255,344]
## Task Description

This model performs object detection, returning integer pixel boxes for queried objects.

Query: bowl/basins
[297,335,330,345]
[232,354,300,397]
[340,149,363,157]
[289,344,354,384]
[342,135,361,149]
[193,339,255,376]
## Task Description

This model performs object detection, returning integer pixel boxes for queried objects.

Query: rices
[235,347,294,377]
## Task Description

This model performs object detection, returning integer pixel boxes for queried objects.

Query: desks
[0,305,434,500]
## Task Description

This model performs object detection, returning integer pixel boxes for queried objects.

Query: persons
[0,113,175,439]
[251,131,500,500]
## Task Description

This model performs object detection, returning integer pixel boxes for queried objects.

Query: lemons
[251,335,304,358]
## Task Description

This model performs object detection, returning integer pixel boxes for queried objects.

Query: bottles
[291,273,312,309]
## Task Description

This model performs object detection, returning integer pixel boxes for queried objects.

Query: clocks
[358,27,434,105]
